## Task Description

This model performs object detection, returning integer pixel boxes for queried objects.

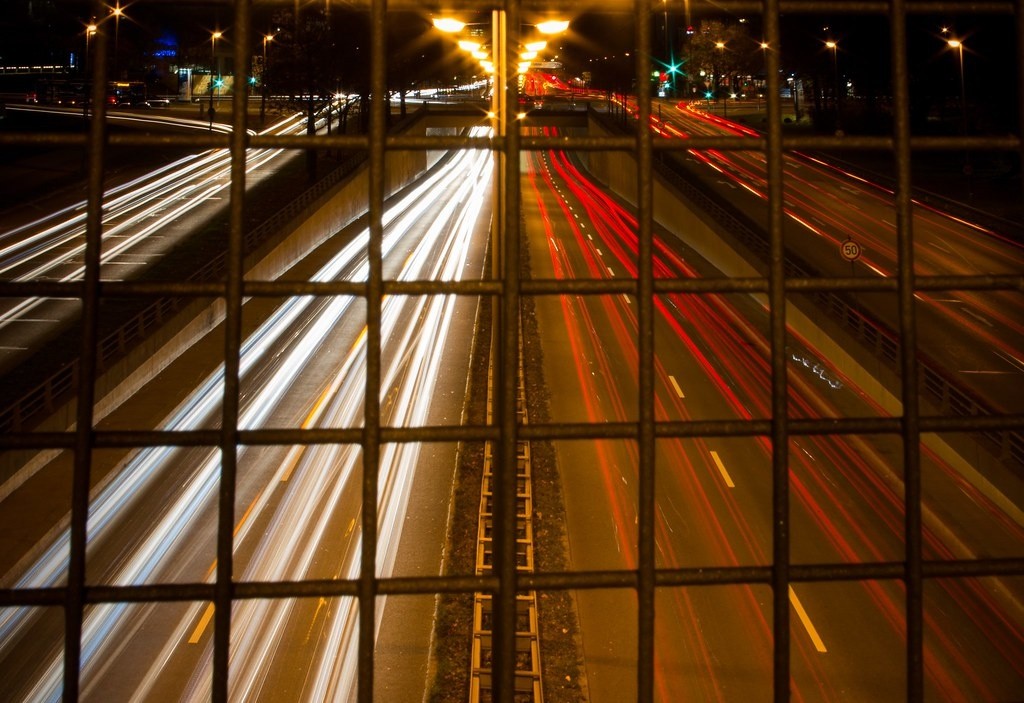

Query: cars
[0,68,171,115]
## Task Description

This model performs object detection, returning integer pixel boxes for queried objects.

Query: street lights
[208,30,224,134]
[715,41,728,118]
[259,35,276,126]
[80,24,99,121]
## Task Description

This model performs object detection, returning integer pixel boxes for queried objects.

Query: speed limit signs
[841,239,861,262]
